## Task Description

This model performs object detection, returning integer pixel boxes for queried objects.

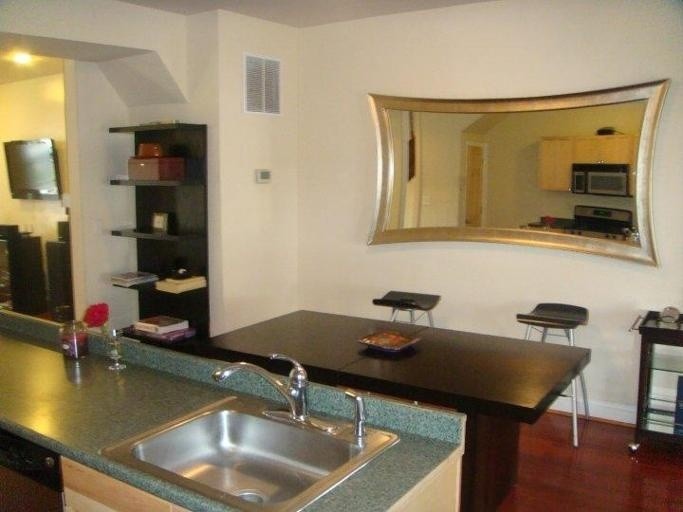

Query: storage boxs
[126,156,186,182]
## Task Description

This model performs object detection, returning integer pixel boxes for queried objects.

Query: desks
[207,308,590,512]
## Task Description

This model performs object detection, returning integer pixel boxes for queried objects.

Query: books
[155,275,207,294]
[135,314,197,341]
[111,272,160,288]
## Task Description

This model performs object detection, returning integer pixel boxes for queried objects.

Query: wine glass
[104,329,128,370]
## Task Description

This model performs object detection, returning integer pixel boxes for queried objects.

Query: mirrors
[364,77,672,270]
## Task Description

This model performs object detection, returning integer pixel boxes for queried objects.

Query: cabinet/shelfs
[626,311,682,454]
[534,135,637,198]
[106,123,205,350]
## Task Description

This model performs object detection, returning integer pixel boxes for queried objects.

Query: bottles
[62,320,87,360]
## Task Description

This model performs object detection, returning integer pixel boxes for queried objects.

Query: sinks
[96,396,400,512]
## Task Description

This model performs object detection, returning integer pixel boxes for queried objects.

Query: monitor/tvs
[4,138,62,202]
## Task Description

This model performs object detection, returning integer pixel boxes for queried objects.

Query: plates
[357,331,421,353]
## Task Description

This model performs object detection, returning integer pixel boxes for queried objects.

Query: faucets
[213,352,308,417]
[343,390,368,437]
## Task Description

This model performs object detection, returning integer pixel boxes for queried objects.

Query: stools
[372,288,590,449]
